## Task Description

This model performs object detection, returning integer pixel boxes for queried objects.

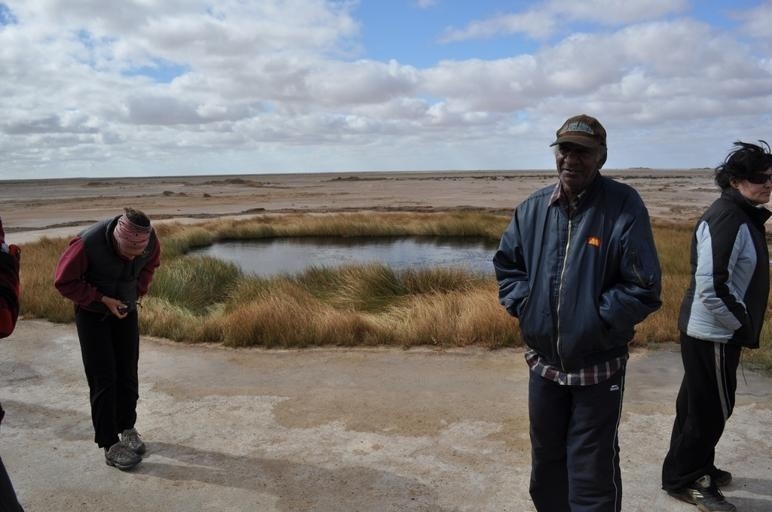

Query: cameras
[119,302,137,312]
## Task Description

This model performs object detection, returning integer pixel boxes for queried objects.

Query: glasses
[745,172,772,184]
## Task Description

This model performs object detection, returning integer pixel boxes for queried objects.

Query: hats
[549,114,606,148]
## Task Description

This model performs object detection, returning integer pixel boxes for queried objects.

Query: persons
[492,113,665,510]
[53,205,160,472]
[0,220,28,511]
[661,137,771,511]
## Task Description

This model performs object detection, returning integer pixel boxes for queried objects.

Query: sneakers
[105,427,146,471]
[662,465,737,512]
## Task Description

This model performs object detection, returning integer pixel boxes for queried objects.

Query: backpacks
[0,220,22,339]
